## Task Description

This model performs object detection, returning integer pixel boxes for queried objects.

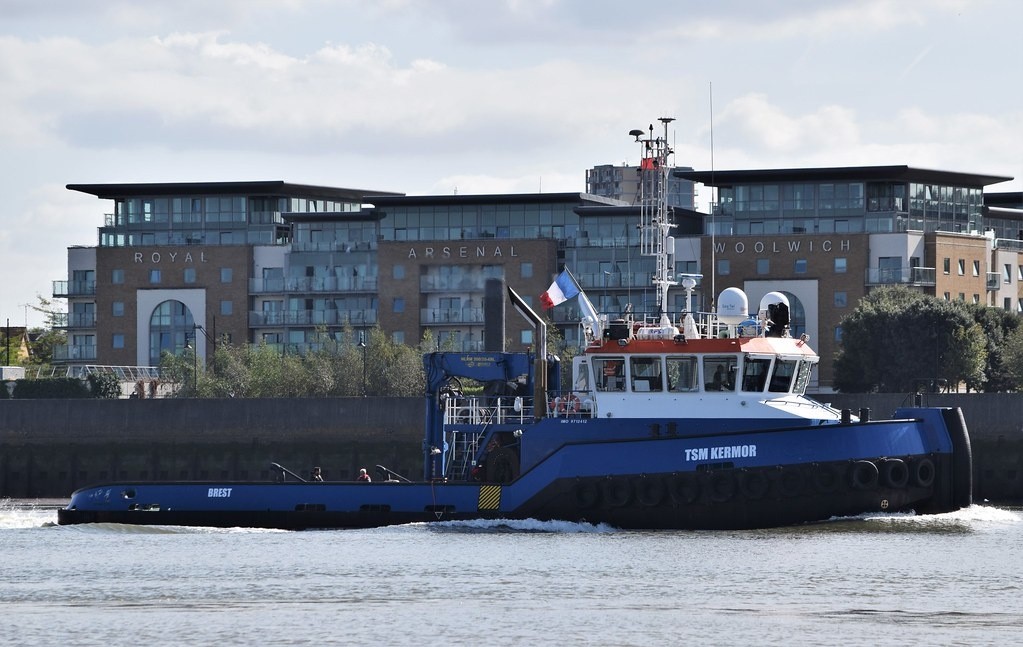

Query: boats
[55,116,976,534]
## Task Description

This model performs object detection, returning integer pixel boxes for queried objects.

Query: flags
[539,270,580,310]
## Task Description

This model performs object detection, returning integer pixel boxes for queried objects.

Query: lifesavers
[668,472,704,506]
[570,480,602,510]
[910,458,935,488]
[807,461,838,495]
[635,477,667,507]
[601,478,632,507]
[705,469,739,503]
[738,469,770,500]
[561,395,582,414]
[882,458,911,489]
[847,459,879,494]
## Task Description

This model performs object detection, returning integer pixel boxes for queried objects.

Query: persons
[713,364,728,390]
[310,467,323,481]
[357,469,371,482]
